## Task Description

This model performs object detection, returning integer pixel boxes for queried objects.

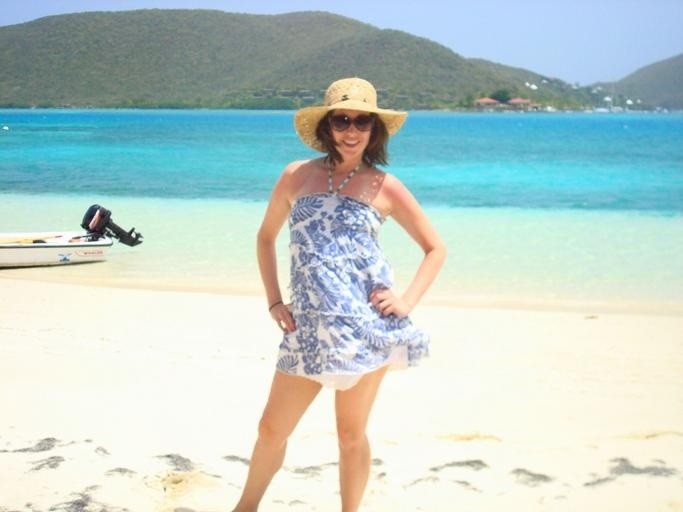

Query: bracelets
[269,298,283,311]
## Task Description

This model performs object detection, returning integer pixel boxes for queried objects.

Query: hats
[293,78,408,152]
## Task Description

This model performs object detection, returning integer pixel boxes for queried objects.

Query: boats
[0,204,144,269]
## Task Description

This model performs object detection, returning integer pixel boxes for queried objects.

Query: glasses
[327,110,378,133]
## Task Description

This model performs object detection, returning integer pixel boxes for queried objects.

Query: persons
[233,77,447,512]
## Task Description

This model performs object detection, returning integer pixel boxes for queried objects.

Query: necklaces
[325,163,365,195]
